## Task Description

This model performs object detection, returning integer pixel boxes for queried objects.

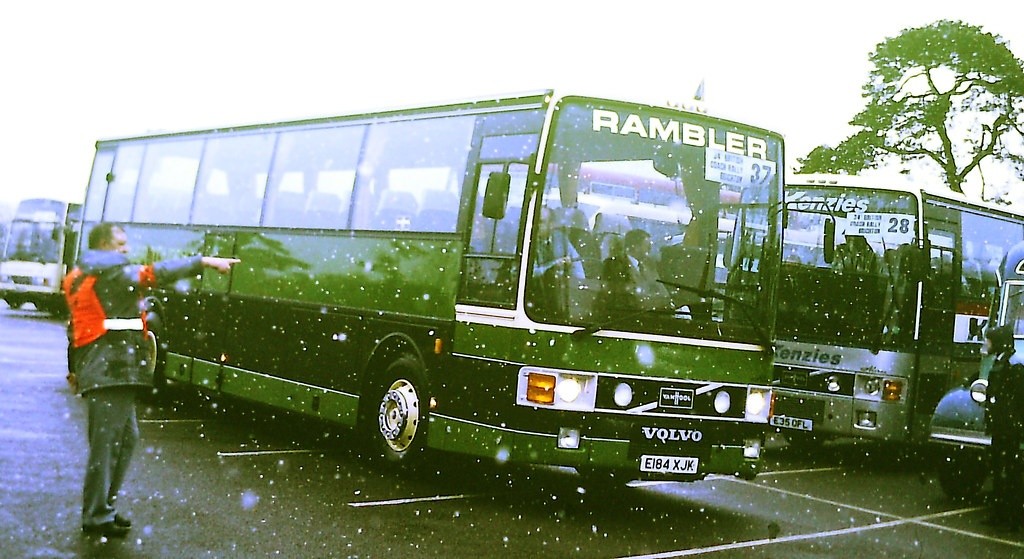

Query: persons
[510,203,586,287]
[595,226,678,317]
[978,325,1024,533]
[62,222,243,540]
[822,235,952,277]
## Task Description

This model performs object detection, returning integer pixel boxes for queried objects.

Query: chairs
[147,186,652,306]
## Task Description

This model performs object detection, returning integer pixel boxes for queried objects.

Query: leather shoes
[112,515,131,529]
[81,521,133,538]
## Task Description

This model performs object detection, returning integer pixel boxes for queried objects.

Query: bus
[0,199,86,321]
[66,86,840,517]
[713,183,1019,466]
[925,236,1024,520]
